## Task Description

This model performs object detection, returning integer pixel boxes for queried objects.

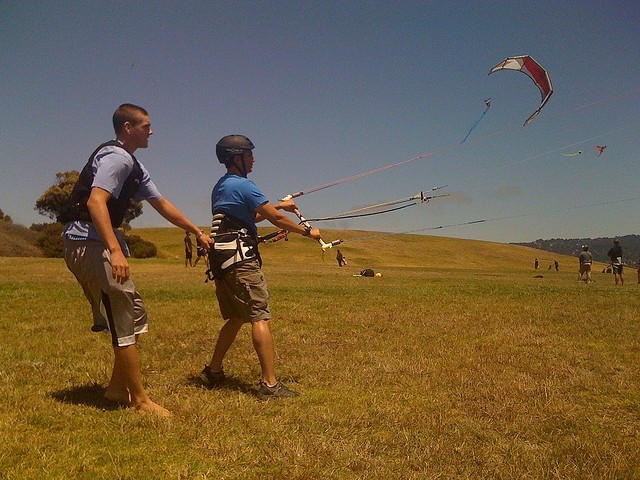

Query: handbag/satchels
[361,269,374,277]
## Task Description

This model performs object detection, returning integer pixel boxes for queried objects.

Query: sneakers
[257,378,300,399]
[195,364,227,390]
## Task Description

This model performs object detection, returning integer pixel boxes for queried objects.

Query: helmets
[215,135,255,163]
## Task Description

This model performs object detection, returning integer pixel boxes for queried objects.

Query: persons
[577,245,593,283]
[60,103,214,416]
[199,135,322,400]
[184,230,195,268]
[193,238,208,268]
[607,240,624,286]
[336,250,345,267]
[533,257,559,272]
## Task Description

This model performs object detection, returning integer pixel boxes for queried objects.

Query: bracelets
[195,229,204,239]
[302,227,310,237]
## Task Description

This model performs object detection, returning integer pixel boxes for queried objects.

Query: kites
[560,151,582,156]
[457,98,492,144]
[593,144,608,158]
